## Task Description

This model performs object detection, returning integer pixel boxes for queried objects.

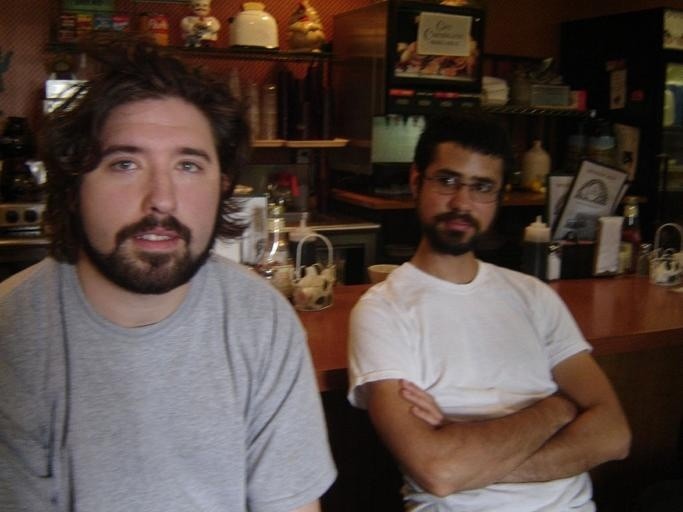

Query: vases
[523,140,552,184]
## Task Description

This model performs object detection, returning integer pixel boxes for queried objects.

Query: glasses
[420,174,504,203]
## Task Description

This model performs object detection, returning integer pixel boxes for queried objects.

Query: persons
[177,0,222,50]
[343,108,635,511]
[3,52,339,510]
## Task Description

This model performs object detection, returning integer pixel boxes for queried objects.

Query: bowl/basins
[369,264,404,285]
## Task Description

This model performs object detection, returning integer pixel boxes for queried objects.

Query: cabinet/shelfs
[44,40,350,211]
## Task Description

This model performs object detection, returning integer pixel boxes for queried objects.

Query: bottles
[521,140,550,191]
[520,216,562,281]
[256,206,296,305]
[288,217,318,279]
[621,196,642,277]
[225,65,279,140]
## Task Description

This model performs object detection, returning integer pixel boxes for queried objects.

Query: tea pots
[0,115,41,204]
